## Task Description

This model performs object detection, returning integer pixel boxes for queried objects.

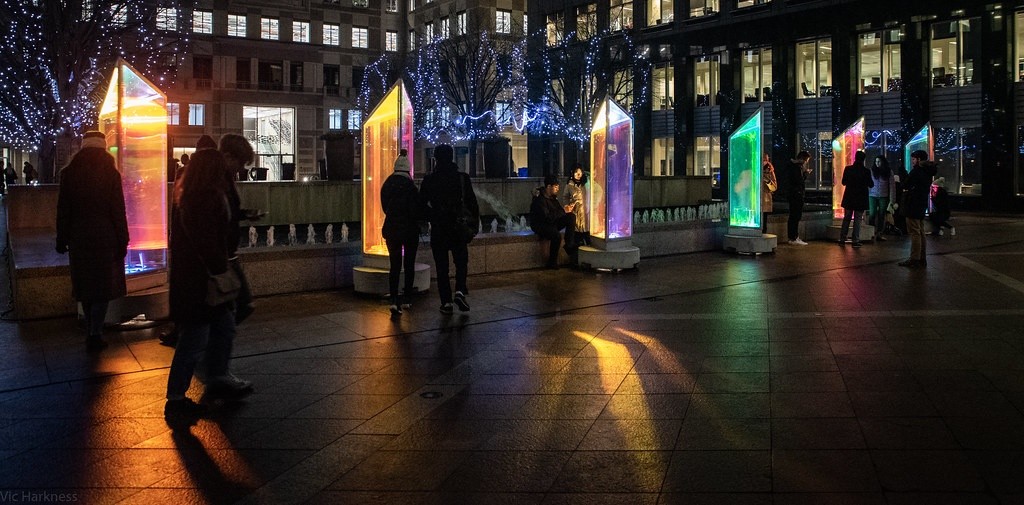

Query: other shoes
[85,336,107,352]
[208,370,253,389]
[877,237,887,241]
[833,238,845,247]
[439,304,453,314]
[163,398,209,422]
[852,242,863,248]
[788,238,808,246]
[455,294,472,311]
[898,257,926,270]
[390,302,403,314]
[235,301,256,325]
[937,226,944,236]
[160,329,180,348]
[401,296,412,308]
[550,261,559,270]
[951,228,956,237]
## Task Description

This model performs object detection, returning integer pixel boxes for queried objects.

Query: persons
[55,132,130,353]
[0,161,18,196]
[869,155,896,242]
[159,133,262,415]
[931,177,956,236]
[836,152,874,248]
[762,152,777,233]
[417,144,479,313]
[530,176,581,268]
[563,163,590,246]
[381,149,432,314]
[23,162,53,184]
[786,152,811,246]
[898,150,937,268]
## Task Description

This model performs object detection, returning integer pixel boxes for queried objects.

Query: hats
[393,149,411,171]
[934,177,945,189]
[80,131,106,149]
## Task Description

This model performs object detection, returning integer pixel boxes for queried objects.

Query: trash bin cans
[281,163,295,180]
[239,169,248,181]
[518,168,528,177]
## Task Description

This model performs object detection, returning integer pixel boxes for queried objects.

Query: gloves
[117,244,129,260]
[55,236,69,254]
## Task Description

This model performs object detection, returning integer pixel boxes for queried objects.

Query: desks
[869,84,881,93]
[820,85,832,96]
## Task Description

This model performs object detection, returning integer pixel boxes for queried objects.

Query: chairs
[861,78,869,94]
[871,76,880,84]
[755,87,772,100]
[933,66,954,86]
[801,81,816,96]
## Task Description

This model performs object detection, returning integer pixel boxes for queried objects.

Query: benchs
[702,7,717,14]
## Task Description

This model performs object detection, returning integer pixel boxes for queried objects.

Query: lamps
[99,55,170,284]
[359,77,419,271]
[726,104,764,235]
[588,93,635,246]
[831,114,868,221]
[905,121,935,215]
